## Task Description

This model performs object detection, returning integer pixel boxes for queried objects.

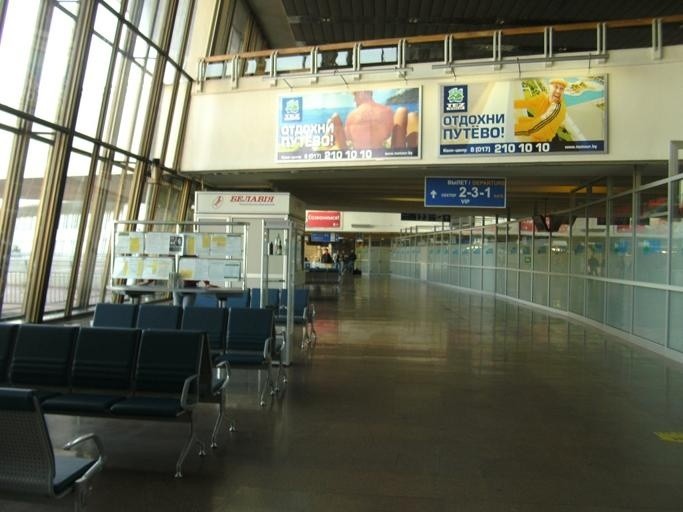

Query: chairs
[0,387,108,511]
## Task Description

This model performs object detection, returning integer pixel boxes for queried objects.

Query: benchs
[0,323,237,479]
[194,288,317,350]
[91,302,289,405]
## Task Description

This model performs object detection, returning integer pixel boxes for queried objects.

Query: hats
[549,79,568,87]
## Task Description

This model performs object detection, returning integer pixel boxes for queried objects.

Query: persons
[331,88,418,152]
[321,246,357,276]
[513,78,585,143]
